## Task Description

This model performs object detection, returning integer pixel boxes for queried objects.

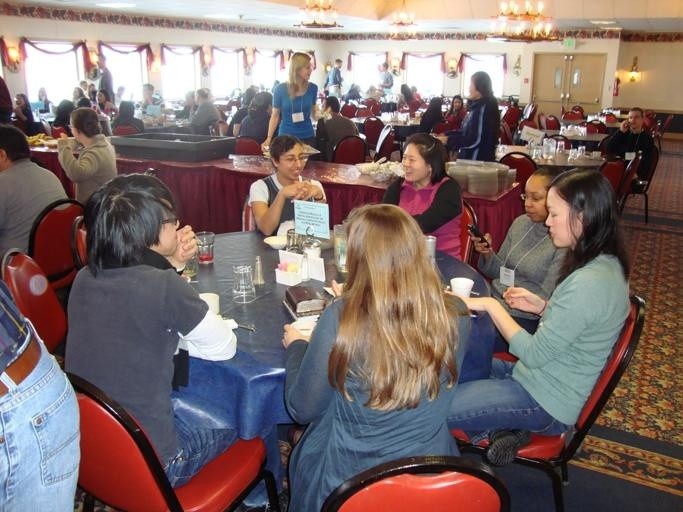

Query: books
[283,286,330,324]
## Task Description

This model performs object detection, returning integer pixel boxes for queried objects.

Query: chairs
[65,373,281,512]
[0,86,676,280]
[320,455,513,512]
[450,294,645,509]
[3,197,554,470]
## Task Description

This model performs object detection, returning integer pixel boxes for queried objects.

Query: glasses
[519,192,545,202]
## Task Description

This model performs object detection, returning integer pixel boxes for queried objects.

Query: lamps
[485,0,563,46]
[291,1,344,32]
[626,55,643,85]
[388,0,418,27]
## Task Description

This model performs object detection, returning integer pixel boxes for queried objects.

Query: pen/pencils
[298,175,303,183]
[238,324,256,332]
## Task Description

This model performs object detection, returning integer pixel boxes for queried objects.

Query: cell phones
[467,222,491,249]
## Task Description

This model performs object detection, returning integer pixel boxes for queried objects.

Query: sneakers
[484,428,532,467]
[277,428,308,469]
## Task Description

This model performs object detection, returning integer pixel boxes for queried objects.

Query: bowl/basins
[262,235,286,251]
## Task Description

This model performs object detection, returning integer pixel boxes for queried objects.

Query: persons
[603,107,659,192]
[443,164,630,476]
[383,133,463,261]
[1,283,81,511]
[248,136,327,237]
[261,53,331,153]
[1,52,166,138]
[469,164,572,353]
[459,72,500,161]
[419,95,466,134]
[2,125,68,255]
[281,205,471,512]
[174,80,279,144]
[317,59,423,157]
[57,107,116,204]
[65,173,287,512]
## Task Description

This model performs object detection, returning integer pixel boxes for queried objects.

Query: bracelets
[537,301,546,315]
[314,195,323,201]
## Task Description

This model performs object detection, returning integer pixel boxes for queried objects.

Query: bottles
[275,230,322,286]
[250,256,266,286]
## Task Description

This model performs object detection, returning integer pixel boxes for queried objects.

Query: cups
[556,140,565,153]
[229,264,256,303]
[568,145,586,161]
[134,113,164,126]
[427,235,436,268]
[380,111,409,123]
[449,277,474,297]
[332,224,348,272]
[184,256,200,276]
[527,138,555,159]
[195,231,215,264]
[558,124,586,137]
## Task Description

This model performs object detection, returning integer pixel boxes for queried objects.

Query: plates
[448,158,518,198]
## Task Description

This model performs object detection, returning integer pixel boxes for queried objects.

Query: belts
[0,325,42,398]
